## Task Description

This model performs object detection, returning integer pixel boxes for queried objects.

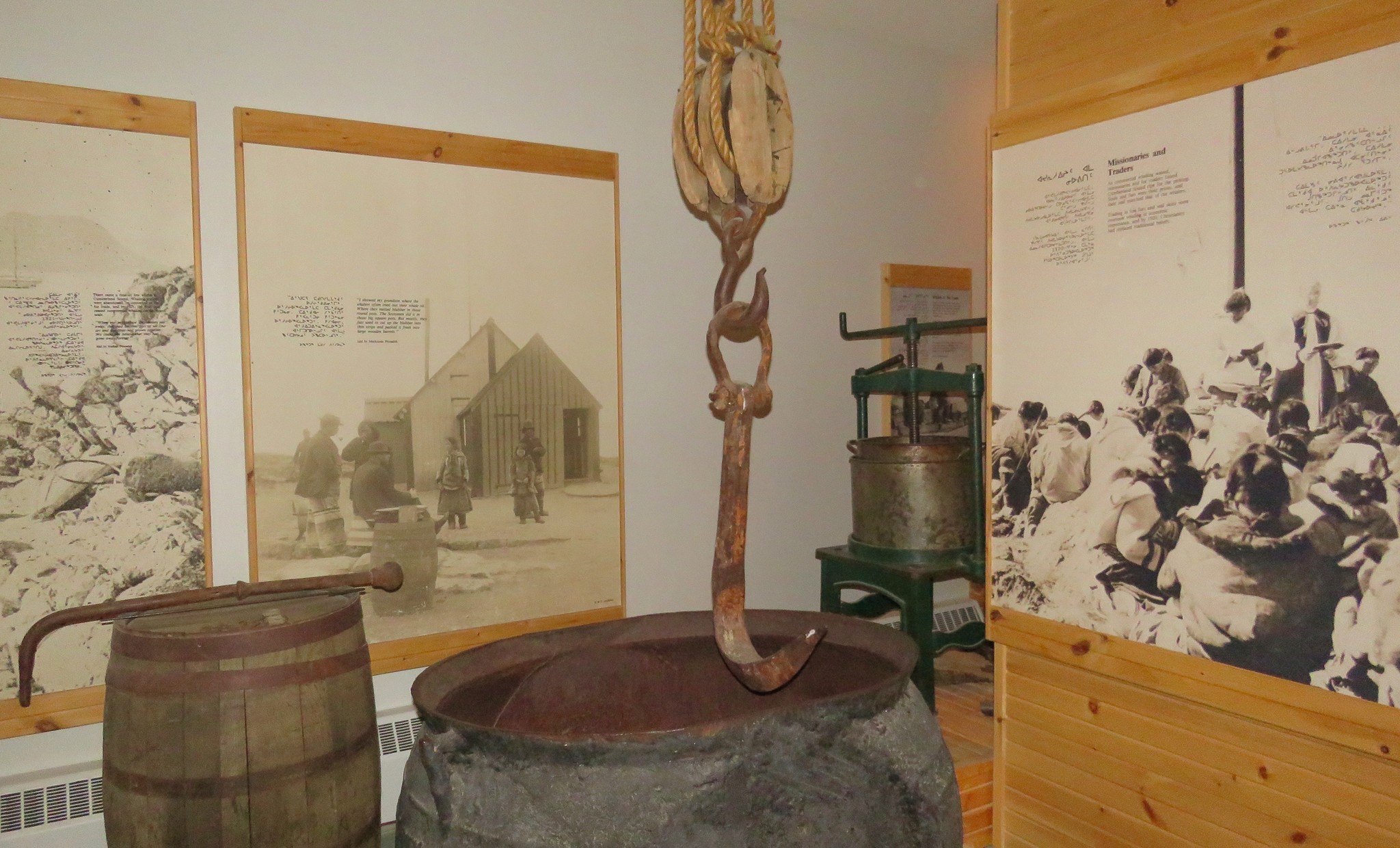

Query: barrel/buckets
[396,608,963,848]
[103,592,381,848]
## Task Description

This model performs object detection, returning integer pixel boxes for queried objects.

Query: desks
[816,545,985,716]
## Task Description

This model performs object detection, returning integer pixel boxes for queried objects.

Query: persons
[292,414,550,544]
[993,285,1400,708]
